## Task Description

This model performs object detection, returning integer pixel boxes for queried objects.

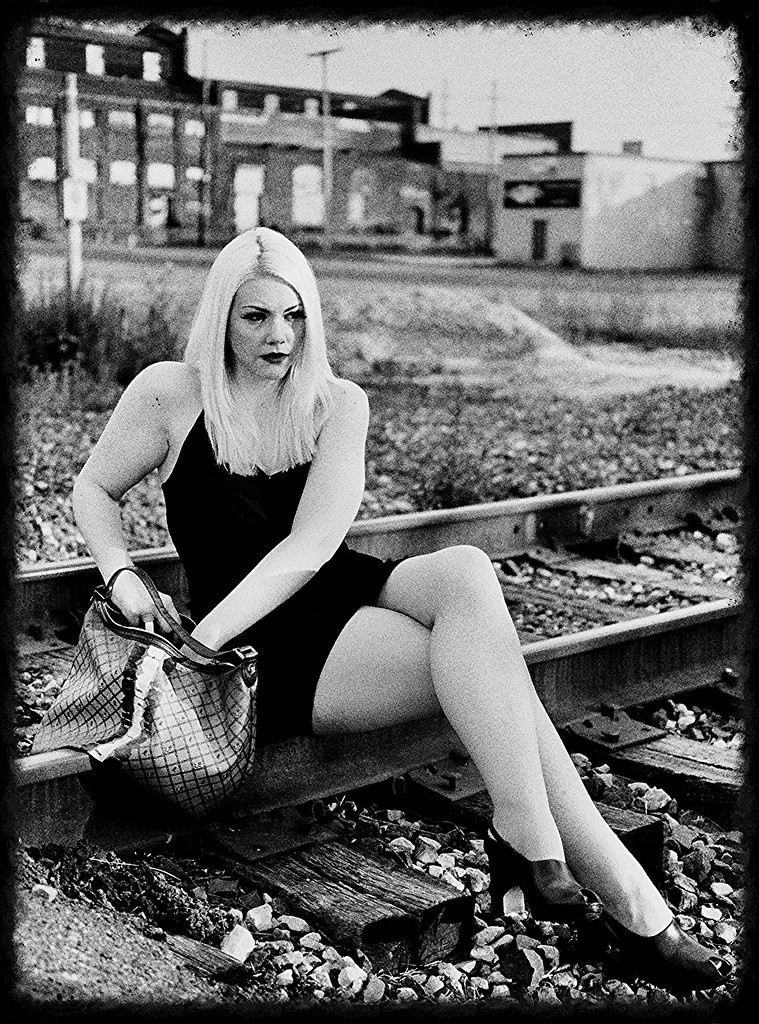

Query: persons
[70,225,735,994]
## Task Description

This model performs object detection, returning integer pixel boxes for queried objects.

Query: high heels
[482,818,603,923]
[591,907,732,995]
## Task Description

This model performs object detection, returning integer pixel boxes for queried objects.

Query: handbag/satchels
[31,566,261,813]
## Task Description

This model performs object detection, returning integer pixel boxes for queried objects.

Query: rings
[141,613,155,622]
